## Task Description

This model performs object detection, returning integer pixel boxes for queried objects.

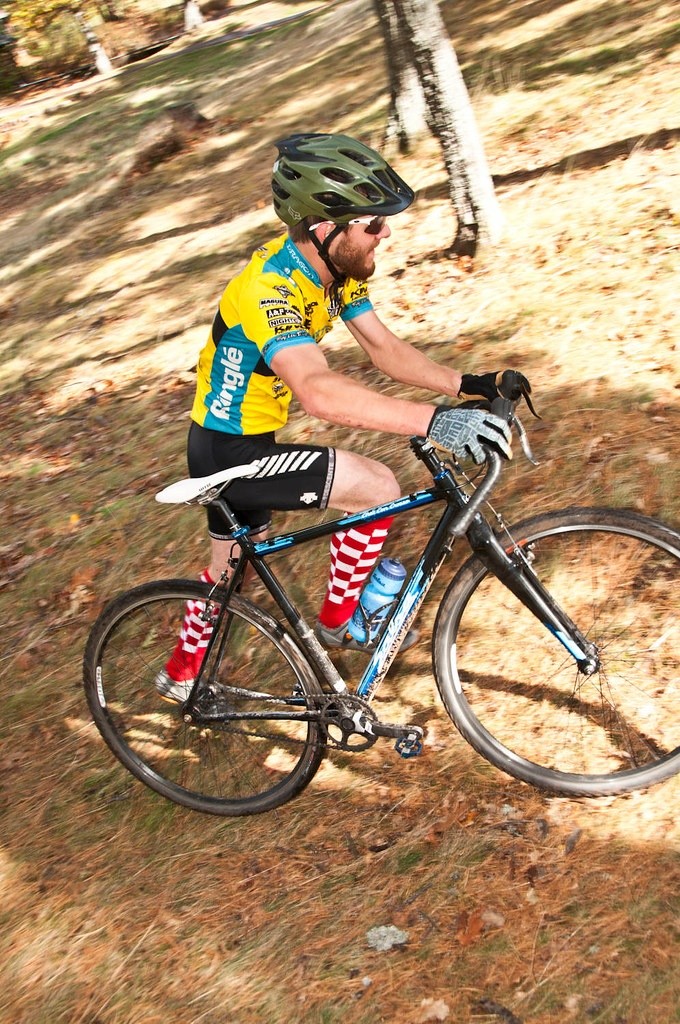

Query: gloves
[426,399,514,465]
[456,368,533,409]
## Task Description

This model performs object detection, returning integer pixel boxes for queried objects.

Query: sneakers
[154,666,226,721]
[312,614,420,655]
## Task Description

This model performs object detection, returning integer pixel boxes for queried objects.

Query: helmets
[269,132,415,227]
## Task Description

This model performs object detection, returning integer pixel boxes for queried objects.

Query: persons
[157,155,533,709]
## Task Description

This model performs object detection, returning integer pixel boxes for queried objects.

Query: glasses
[308,216,386,234]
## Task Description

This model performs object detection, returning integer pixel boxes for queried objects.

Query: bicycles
[78,369,680,819]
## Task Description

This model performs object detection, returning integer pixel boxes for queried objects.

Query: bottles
[347,554,408,645]
